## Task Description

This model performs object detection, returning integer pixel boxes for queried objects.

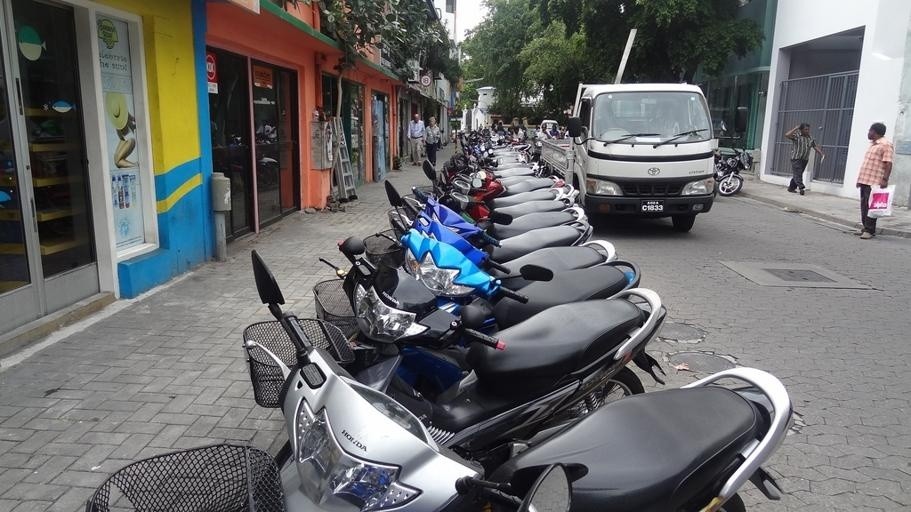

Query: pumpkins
[328,114,358,202]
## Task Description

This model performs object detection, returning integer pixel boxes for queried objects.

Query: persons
[853,123,894,239]
[537,123,552,142]
[533,126,540,136]
[109,93,139,169]
[549,124,560,137]
[407,114,425,166]
[785,123,823,195]
[521,117,528,129]
[494,122,499,131]
[424,117,441,166]
[497,122,505,131]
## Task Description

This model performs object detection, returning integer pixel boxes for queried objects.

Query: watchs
[310,235,640,399]
[363,195,592,275]
[243,230,667,479]
[715,146,752,196]
[381,178,618,349]
[87,244,793,512]
[410,127,585,238]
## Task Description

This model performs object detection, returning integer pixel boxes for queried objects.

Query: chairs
[799,189,804,195]
[787,188,797,193]
[852,230,878,241]
[412,161,422,166]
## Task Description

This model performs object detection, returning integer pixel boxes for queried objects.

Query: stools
[411,136,426,140]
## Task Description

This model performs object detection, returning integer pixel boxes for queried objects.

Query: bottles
[106,92,129,130]
